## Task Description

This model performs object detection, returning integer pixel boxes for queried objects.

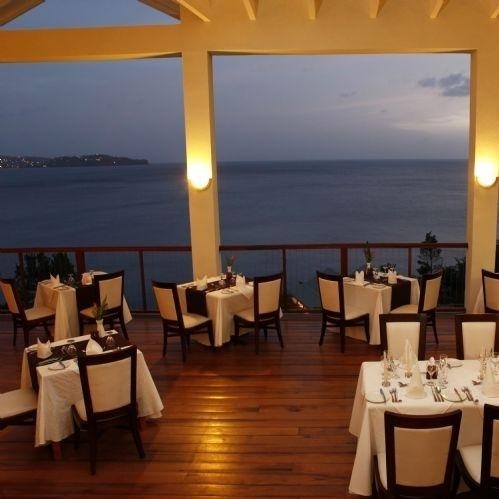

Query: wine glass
[67,340,77,356]
[361,342,499,379]
[106,332,116,348]
[56,269,95,290]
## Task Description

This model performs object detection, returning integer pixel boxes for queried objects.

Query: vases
[226,265,233,283]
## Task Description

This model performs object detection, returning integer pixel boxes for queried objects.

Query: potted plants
[363,241,375,276]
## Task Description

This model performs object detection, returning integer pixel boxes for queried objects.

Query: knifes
[361,379,481,408]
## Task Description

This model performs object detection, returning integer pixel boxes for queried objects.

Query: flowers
[225,255,237,266]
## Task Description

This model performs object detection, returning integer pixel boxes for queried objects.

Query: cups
[354,262,401,287]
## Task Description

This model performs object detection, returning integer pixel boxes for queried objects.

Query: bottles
[90,330,100,342]
